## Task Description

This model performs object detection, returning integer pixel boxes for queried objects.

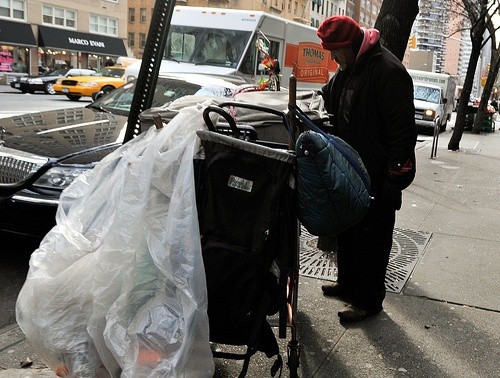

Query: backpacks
[194,144,293,377]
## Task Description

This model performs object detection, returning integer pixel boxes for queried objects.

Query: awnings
[37,24,128,57]
[0,19,38,48]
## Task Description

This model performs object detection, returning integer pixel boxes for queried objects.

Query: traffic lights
[481,77,487,87]
[408,35,416,48]
[486,63,491,72]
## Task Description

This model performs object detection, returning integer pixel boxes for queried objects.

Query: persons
[488,99,500,130]
[468,97,480,106]
[317,16,418,320]
[454,96,460,112]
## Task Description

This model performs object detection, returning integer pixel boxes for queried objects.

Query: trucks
[404,68,457,135]
[124,6,339,100]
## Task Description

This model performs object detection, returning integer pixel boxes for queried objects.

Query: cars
[52,56,137,103]
[10,68,97,95]
[0,73,287,247]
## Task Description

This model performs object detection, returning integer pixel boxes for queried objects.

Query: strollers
[172,101,305,378]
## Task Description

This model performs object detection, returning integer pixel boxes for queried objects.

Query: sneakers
[338,303,382,321]
[322,283,342,295]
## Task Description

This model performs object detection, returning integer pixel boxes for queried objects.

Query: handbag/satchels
[285,103,371,237]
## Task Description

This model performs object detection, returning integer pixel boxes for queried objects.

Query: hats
[317,16,361,50]
